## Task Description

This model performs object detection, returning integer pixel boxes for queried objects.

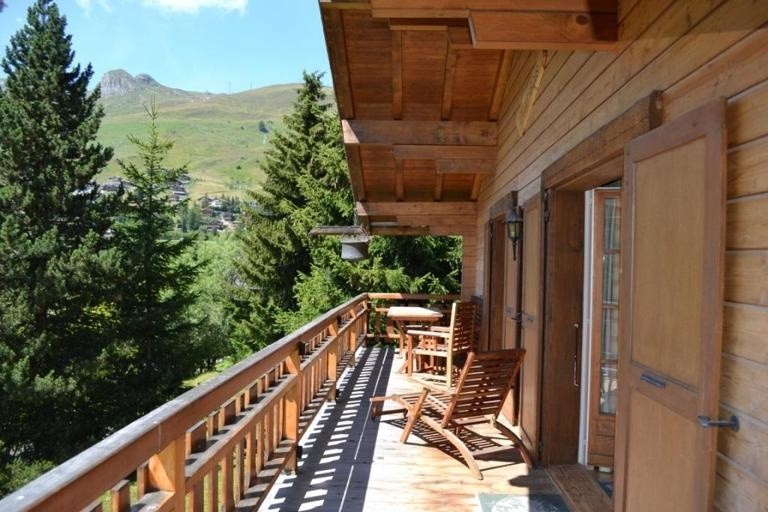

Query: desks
[386,305,444,359]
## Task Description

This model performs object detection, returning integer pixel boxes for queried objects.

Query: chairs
[407,299,478,387]
[365,347,536,482]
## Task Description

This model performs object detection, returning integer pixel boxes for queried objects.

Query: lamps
[504,206,522,262]
